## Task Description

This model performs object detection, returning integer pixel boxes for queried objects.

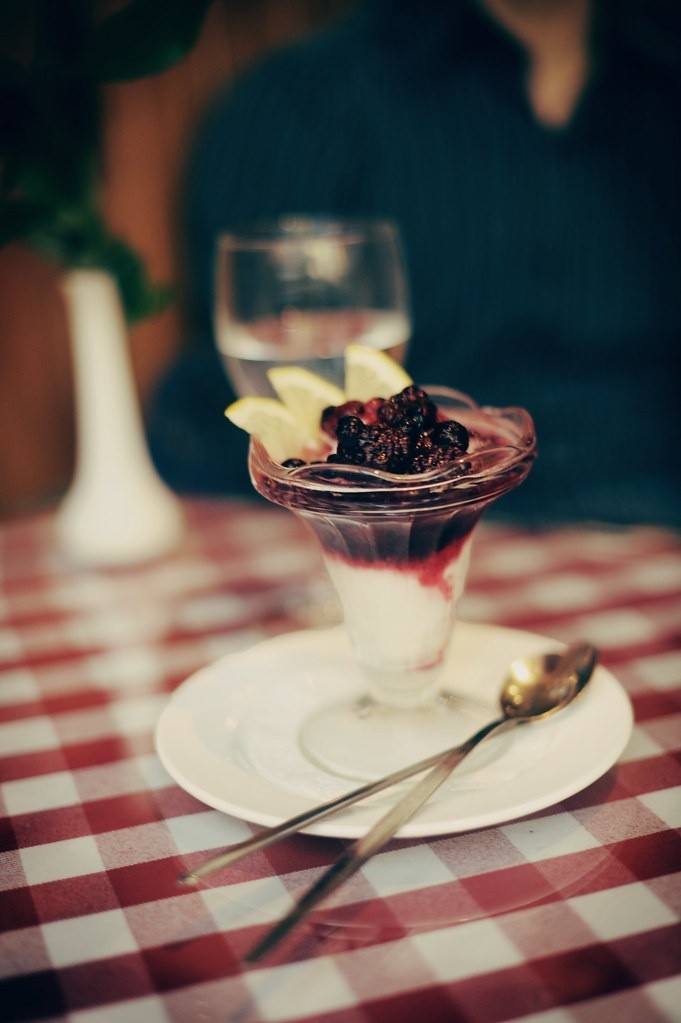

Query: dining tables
[0,495,681,1023]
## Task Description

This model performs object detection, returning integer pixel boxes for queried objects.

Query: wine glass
[213,216,415,622]
[246,385,537,783]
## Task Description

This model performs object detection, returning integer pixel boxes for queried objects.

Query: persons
[0,0,205,332]
[146,0,681,528]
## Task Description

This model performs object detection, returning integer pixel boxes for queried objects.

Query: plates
[155,622,635,838]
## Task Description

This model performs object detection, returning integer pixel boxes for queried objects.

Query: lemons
[267,366,344,426]
[224,396,337,464]
[344,344,412,401]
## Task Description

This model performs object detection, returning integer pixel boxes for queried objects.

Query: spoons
[177,644,598,887]
[241,654,577,968]
